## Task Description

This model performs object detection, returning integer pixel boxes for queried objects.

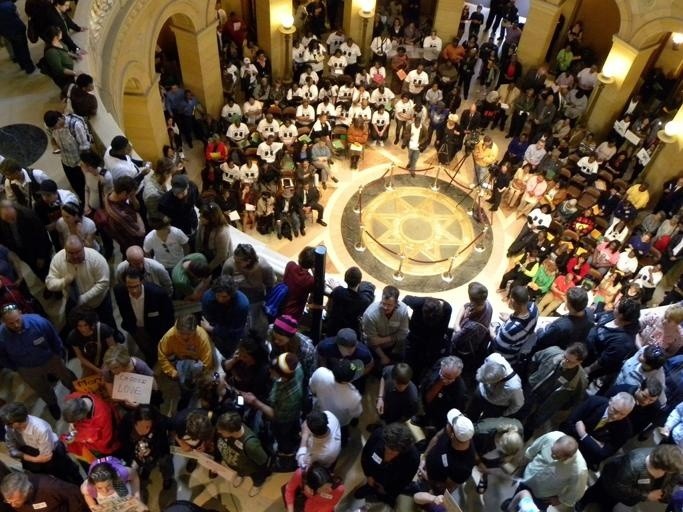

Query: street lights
[357,0,375,73]
[628,121,678,190]
[580,63,615,135]
[278,14,297,90]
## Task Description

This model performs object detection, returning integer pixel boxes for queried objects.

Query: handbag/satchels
[281,482,307,512]
[67,112,95,144]
[275,452,297,472]
[81,355,101,377]
[27,17,39,43]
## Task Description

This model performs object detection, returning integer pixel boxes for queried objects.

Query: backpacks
[262,264,311,317]
[87,456,128,498]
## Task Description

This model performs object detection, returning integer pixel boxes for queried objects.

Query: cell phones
[237,395,245,406]
[53,150,60,154]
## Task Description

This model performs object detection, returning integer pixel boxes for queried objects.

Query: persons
[0,0,682,512]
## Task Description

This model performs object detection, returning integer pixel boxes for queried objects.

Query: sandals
[477,473,487,494]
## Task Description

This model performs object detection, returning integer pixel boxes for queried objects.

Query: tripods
[456,173,493,225]
[446,150,479,190]
[425,144,451,176]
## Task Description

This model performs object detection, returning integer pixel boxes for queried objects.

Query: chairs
[507,123,663,314]
[214,73,368,225]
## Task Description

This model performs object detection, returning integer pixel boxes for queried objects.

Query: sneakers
[350,137,416,178]
[163,452,262,497]
[486,197,525,303]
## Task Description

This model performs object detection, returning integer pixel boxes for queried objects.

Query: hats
[447,408,475,441]
[36,180,57,195]
[149,212,171,229]
[448,113,458,121]
[171,174,189,193]
[274,314,298,337]
[333,359,365,383]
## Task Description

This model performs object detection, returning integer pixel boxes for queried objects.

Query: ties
[595,418,608,429]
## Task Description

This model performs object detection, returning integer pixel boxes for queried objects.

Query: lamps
[583,62,614,125]
[636,120,678,185]
[278,13,295,87]
[358,0,375,68]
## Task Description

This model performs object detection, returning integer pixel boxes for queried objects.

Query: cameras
[145,162,151,170]
[444,132,457,145]
[466,132,479,146]
[487,162,501,173]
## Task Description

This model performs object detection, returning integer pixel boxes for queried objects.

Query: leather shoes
[278,177,338,241]
